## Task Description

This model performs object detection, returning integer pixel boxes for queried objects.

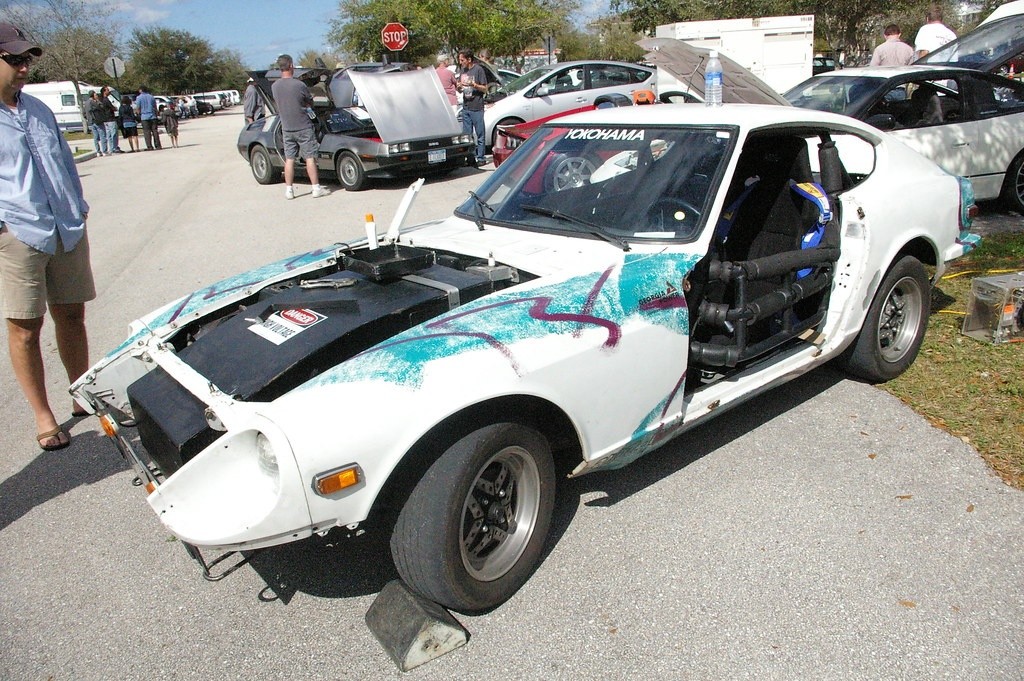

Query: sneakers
[285,190,294,200]
[313,185,331,198]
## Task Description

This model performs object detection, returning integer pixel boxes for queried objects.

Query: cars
[451,60,684,155]
[331,62,411,106]
[59,101,984,615]
[451,68,522,108]
[131,89,241,129]
[237,62,479,192]
[634,0,1024,218]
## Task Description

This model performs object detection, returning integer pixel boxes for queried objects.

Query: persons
[188,96,199,119]
[158,105,179,148]
[85,90,110,156]
[870,24,914,100]
[0,21,96,451]
[914,4,959,95]
[480,48,494,64]
[136,84,162,151]
[243,77,266,127]
[119,96,141,153]
[179,96,187,119]
[435,55,462,113]
[457,48,488,168]
[271,54,331,200]
[99,86,125,153]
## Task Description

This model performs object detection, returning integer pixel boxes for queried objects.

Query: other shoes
[96,153,103,157]
[476,161,486,166]
[115,150,125,153]
[157,147,163,150]
[144,147,153,151]
[103,152,111,156]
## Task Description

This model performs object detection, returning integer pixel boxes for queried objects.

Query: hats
[247,77,254,82]
[437,55,449,64]
[0,22,43,57]
[89,90,95,97]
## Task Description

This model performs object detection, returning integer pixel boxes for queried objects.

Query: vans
[21,80,125,133]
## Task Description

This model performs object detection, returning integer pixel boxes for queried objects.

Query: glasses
[0,53,33,67]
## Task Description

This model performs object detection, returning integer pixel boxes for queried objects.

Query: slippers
[72,397,101,417]
[37,426,70,451]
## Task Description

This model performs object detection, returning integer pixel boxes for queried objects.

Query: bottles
[705,51,724,109]
[363,214,381,251]
[307,107,316,119]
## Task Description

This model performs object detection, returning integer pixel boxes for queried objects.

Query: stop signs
[381,23,410,51]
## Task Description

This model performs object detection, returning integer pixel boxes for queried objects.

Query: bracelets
[473,83,476,87]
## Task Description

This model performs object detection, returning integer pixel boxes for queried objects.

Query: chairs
[837,88,896,114]
[637,131,840,367]
[911,85,946,127]
[634,91,657,104]
[558,69,630,91]
